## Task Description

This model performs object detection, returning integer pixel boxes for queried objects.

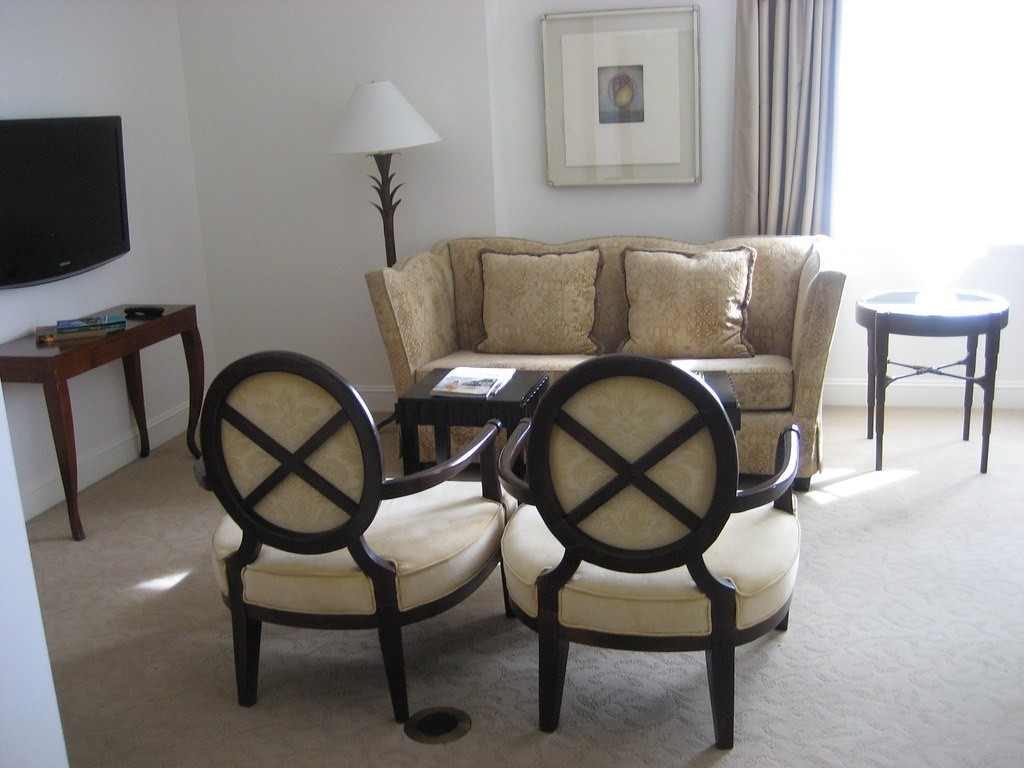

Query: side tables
[856,289,1008,473]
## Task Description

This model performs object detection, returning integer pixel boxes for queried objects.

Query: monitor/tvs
[0,115,131,290]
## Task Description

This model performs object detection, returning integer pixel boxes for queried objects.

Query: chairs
[194,352,518,722]
[497,353,800,749]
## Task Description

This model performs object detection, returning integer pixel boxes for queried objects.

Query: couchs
[366,237,847,491]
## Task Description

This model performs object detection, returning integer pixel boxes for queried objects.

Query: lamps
[322,79,441,456]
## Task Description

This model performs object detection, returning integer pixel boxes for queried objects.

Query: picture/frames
[541,6,701,187]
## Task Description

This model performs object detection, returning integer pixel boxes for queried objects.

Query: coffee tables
[693,371,741,435]
[398,369,548,505]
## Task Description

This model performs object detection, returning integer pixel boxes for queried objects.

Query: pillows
[616,245,756,359]
[471,248,607,354]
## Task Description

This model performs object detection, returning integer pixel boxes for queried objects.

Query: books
[432,367,516,400]
[35,313,126,342]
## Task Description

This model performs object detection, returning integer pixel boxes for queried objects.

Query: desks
[0,305,205,541]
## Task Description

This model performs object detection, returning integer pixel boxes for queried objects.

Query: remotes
[124,306,164,315]
